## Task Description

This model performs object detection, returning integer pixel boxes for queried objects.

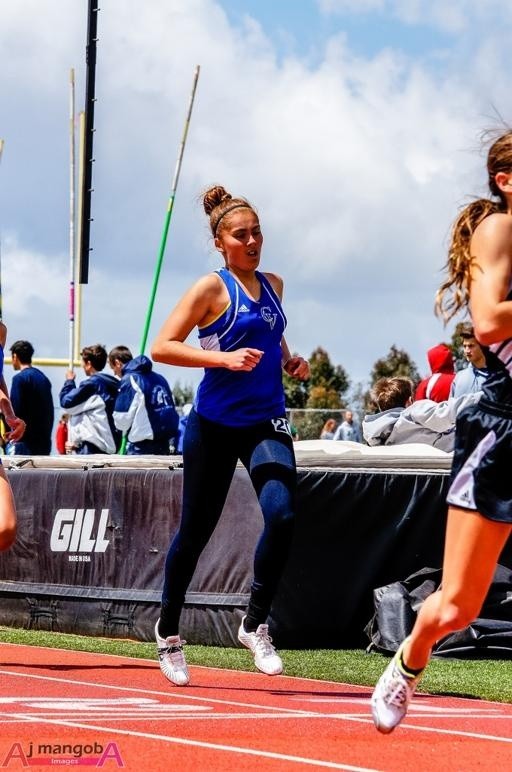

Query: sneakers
[239,615,282,674]
[370,635,434,735]
[154,618,191,686]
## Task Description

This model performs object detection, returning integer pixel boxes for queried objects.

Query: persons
[150,184,312,687]
[370,97,512,735]
[0,319,18,555]
[0,324,489,454]
